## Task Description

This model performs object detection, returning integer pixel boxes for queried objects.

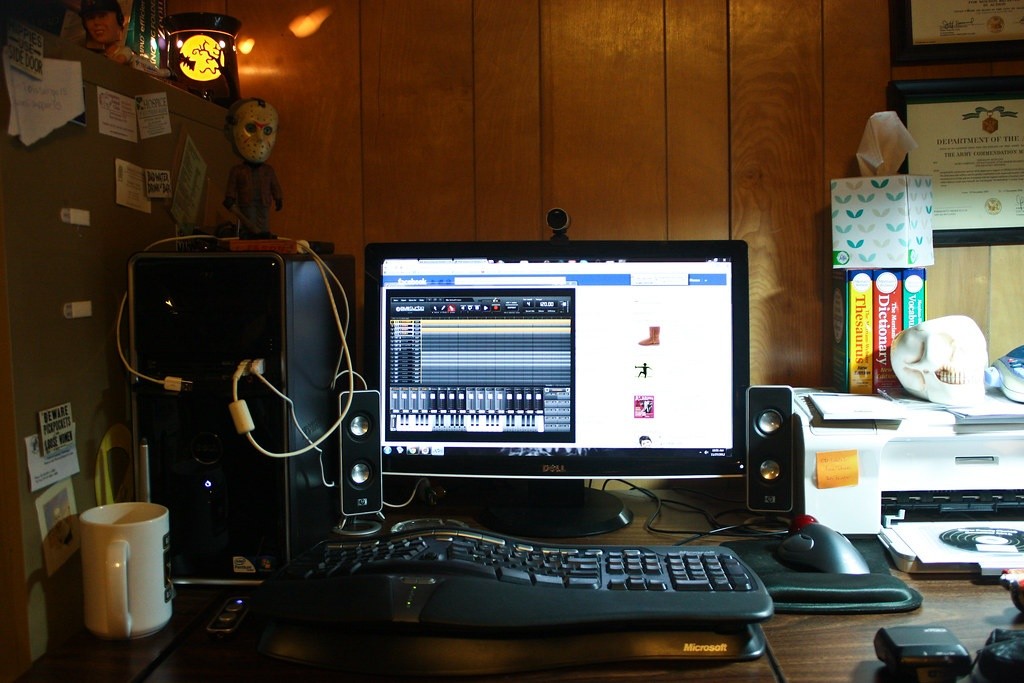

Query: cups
[79,502,171,642]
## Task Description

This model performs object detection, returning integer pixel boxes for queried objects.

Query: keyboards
[255,528,775,630]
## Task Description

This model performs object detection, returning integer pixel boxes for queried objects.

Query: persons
[82,0,125,48]
[224,98,282,239]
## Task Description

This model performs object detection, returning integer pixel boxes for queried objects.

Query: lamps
[163,12,241,109]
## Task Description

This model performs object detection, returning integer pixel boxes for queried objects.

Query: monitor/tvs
[363,240,751,538]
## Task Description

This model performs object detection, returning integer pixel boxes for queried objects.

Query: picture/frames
[887,0,1024,245]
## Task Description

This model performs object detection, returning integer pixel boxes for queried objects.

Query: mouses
[775,515,871,575]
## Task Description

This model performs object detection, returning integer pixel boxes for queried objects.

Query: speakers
[743,385,793,526]
[338,389,383,535]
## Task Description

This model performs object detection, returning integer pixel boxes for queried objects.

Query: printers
[791,387,1024,573]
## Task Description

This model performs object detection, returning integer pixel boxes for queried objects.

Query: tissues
[829,110,935,270]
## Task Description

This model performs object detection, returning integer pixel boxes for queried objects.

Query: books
[823,269,926,395]
[59,0,168,68]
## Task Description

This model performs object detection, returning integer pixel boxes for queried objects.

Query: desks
[20,485,1023,683]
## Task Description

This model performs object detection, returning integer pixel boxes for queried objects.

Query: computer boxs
[126,253,358,584]
